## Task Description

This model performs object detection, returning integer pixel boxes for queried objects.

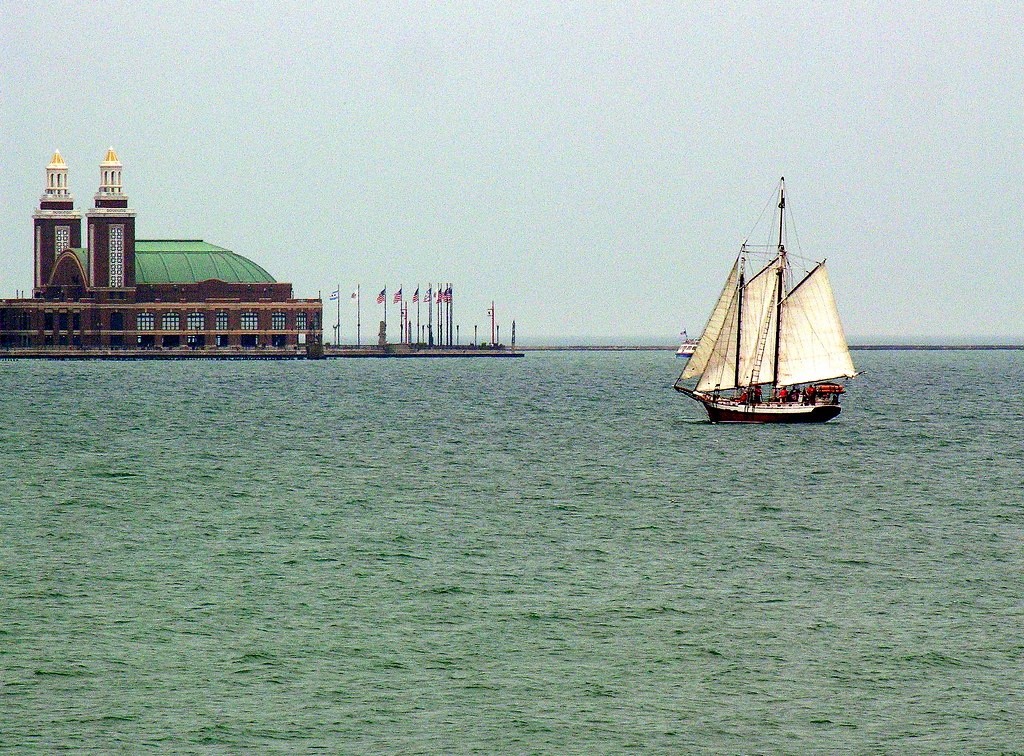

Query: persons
[704,384,840,406]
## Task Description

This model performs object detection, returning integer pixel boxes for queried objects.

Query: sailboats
[675,176,867,424]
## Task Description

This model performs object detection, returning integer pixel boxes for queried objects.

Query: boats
[676,328,699,356]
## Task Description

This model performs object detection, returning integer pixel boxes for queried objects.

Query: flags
[394,288,402,305]
[413,288,418,303]
[376,289,385,305]
[424,287,452,305]
[329,290,339,301]
[351,287,358,299]
